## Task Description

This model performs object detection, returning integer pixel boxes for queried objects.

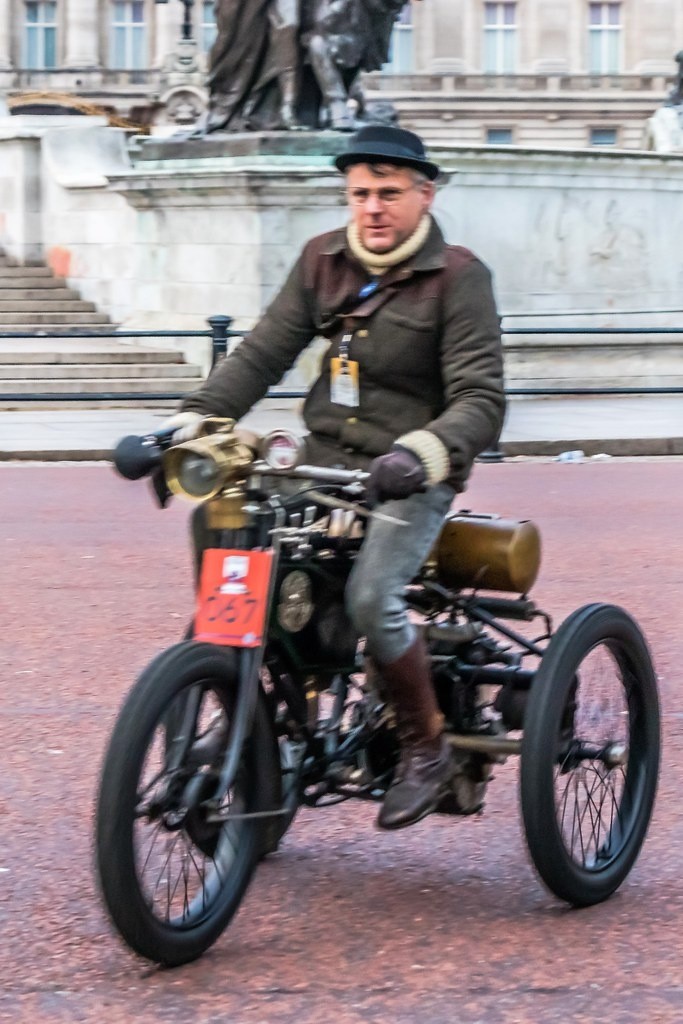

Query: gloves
[152,412,206,446]
[361,447,426,503]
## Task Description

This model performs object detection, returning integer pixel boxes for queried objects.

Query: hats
[335,125,438,180]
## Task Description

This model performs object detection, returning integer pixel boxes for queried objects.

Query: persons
[159,125,507,831]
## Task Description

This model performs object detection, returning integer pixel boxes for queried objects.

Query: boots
[366,624,470,829]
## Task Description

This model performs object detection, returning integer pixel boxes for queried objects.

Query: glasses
[340,179,425,206]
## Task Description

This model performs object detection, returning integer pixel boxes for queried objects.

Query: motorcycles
[93,416,662,969]
